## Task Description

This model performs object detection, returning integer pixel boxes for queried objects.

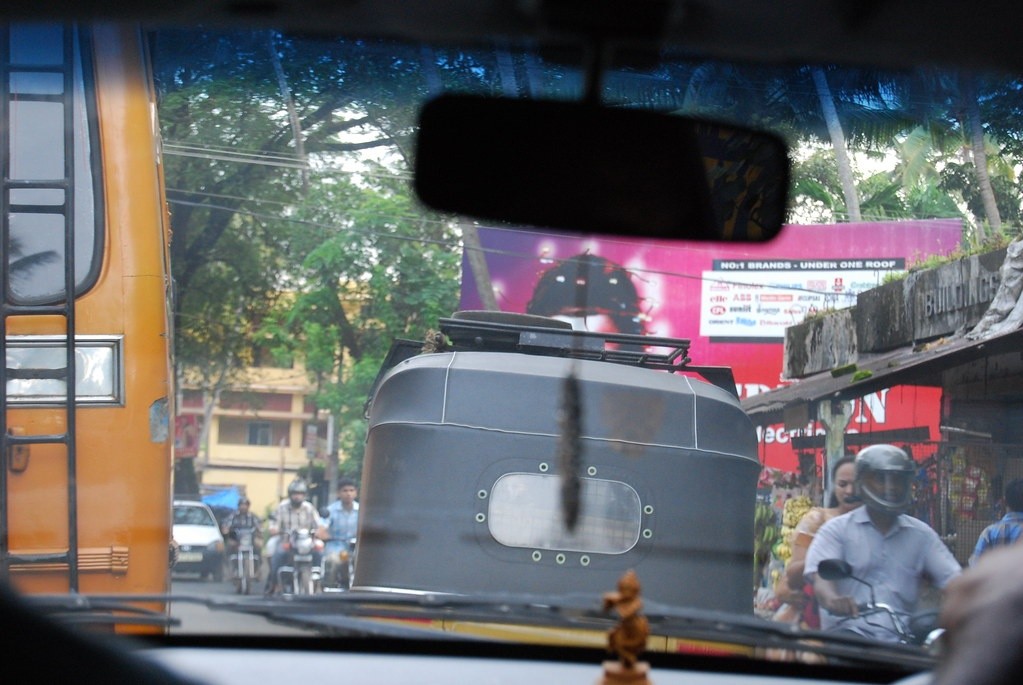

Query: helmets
[288,480,307,504]
[239,500,250,506]
[855,443,916,515]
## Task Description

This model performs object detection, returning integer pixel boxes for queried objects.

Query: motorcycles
[222,524,357,597]
[355,310,761,623]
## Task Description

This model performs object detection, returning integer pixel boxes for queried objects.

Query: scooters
[816,559,945,659]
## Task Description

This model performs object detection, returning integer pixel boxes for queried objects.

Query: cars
[172,495,228,584]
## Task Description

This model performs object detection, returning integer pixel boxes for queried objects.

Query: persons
[605,572,648,664]
[222,498,262,561]
[803,445,963,644]
[263,480,322,594]
[315,480,359,587]
[772,455,859,624]
[969,477,1023,566]
[526,254,644,352]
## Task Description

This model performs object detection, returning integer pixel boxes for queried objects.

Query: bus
[0,0,171,642]
